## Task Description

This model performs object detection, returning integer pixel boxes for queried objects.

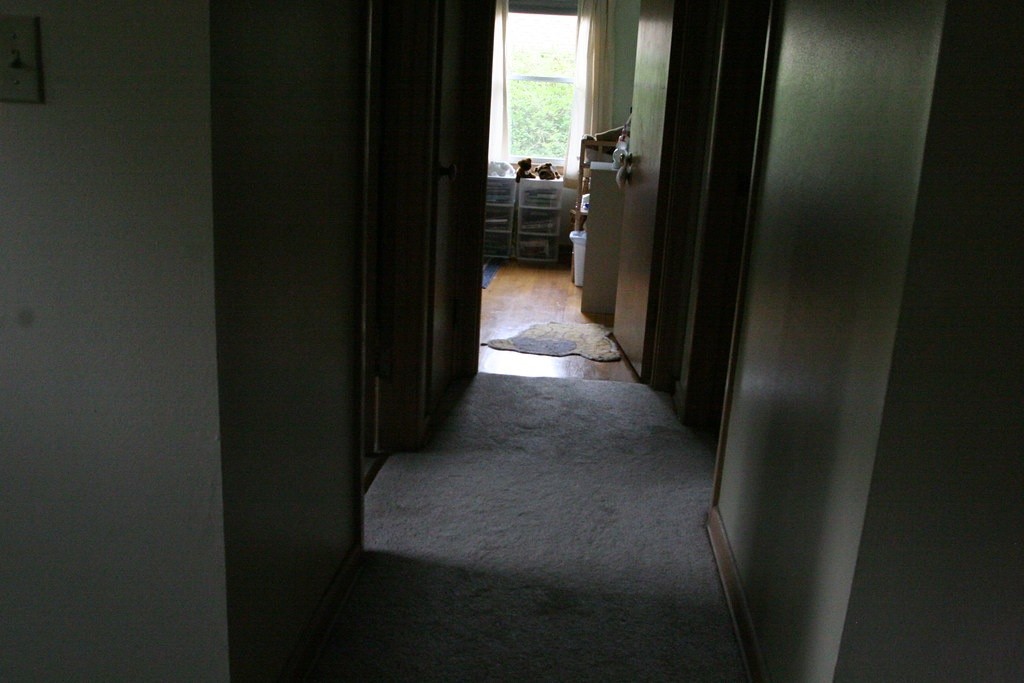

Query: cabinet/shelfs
[580,169,622,315]
[570,140,618,286]
[516,174,564,261]
[483,168,516,263]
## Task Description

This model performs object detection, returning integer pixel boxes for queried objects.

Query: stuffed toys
[535,162,555,180]
[514,158,535,183]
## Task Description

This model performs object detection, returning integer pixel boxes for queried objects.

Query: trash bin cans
[569,230,587,286]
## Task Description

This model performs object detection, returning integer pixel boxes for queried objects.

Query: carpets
[488,321,622,362]
[483,260,504,289]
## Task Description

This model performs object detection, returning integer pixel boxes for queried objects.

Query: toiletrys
[613,128,630,169]
